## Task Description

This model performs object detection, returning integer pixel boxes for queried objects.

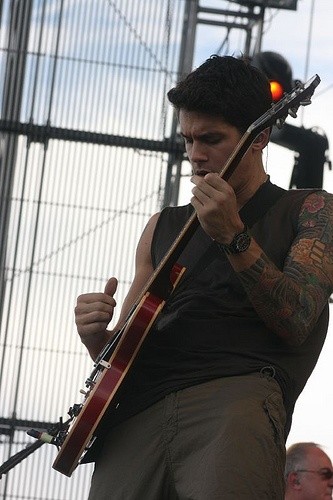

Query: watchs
[218,220,252,256]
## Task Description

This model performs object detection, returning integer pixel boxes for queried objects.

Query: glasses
[296,468,333,478]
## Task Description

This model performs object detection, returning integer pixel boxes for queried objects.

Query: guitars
[52,74,321,478]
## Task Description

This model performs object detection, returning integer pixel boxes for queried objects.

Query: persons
[282,441,333,500]
[72,52,331,500]
